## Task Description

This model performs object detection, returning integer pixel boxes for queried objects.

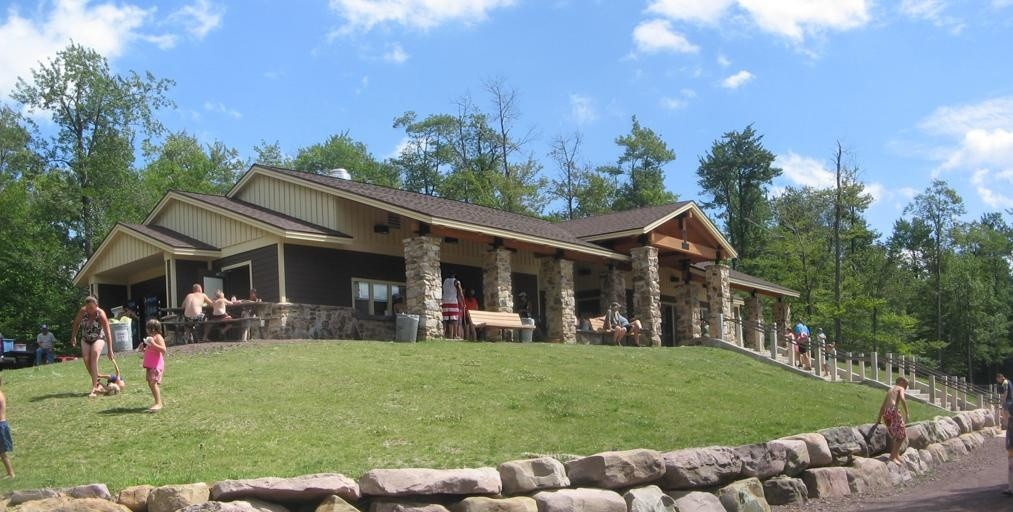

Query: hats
[610,302,621,309]
[42,325,47,328]
[518,292,526,296]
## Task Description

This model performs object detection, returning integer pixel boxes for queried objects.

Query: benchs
[466,309,524,342]
[588,318,642,345]
[159,315,281,342]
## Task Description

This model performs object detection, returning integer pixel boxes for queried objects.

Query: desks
[161,301,273,342]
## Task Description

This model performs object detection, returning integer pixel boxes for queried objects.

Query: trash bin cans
[395,314,420,344]
[520,319,536,343]
[110,324,134,352]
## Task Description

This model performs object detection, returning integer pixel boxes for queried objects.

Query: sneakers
[795,363,811,370]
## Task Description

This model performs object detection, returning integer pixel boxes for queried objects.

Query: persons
[241,287,264,338]
[822,343,832,376]
[393,295,404,314]
[1000,402,1013,497]
[814,327,830,377]
[575,300,643,347]
[136,318,167,412]
[794,317,812,370]
[874,376,911,466]
[442,269,467,340]
[35,323,58,366]
[181,283,215,344]
[96,356,125,396]
[453,279,480,342]
[117,304,141,347]
[995,372,1013,431]
[212,290,233,340]
[0,377,16,480]
[516,290,533,318]
[830,341,836,352]
[71,294,115,397]
[784,327,803,368]
